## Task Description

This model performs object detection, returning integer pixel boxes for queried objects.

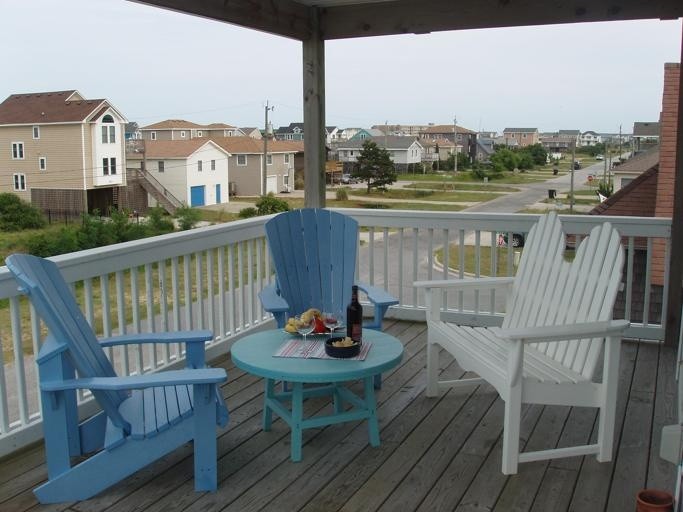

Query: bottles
[346,285,363,346]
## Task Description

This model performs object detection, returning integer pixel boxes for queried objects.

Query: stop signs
[587,176,593,181]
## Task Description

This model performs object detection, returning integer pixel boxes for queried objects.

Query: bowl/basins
[325,337,361,358]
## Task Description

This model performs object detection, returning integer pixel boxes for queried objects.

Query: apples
[308,318,325,333]
[310,320,326,333]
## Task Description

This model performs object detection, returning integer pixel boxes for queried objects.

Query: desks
[231,324,405,462]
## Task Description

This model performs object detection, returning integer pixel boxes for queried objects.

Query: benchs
[412,208,632,475]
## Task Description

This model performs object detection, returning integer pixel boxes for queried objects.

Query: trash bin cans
[554,170,558,175]
[549,190,556,198]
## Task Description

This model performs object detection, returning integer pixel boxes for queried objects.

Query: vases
[635,488,675,512]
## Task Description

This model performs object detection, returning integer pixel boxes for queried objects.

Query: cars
[574,161,581,169]
[596,154,603,160]
[342,174,358,184]
[504,231,529,248]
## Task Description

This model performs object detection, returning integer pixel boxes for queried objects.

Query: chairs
[5,252,230,505]
[258,206,399,394]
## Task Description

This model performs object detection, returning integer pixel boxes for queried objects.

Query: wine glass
[295,314,316,354]
[322,310,344,339]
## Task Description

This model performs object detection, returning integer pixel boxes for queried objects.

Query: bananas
[300,308,321,324]
[285,317,301,333]
